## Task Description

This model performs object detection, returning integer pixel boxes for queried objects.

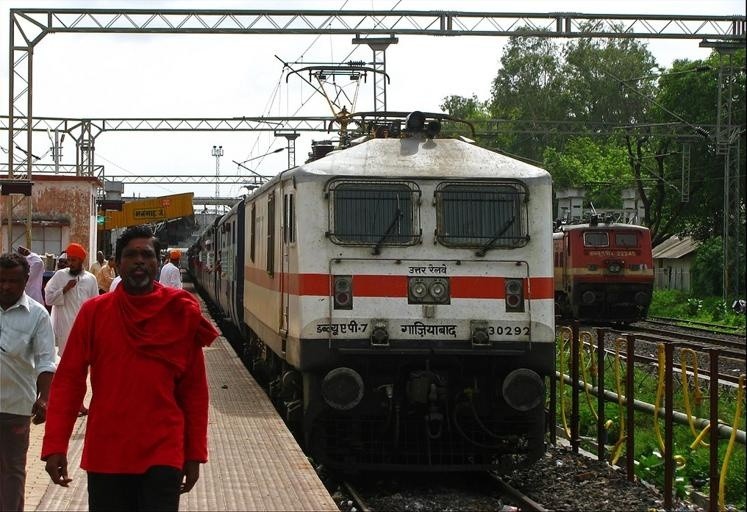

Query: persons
[88,251,108,297]
[158,250,183,291]
[15,245,45,308]
[0,248,60,512]
[95,256,118,296]
[39,223,209,512]
[56,250,69,270]
[42,241,100,417]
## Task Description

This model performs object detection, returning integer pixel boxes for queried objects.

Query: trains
[159,110,559,481]
[553,207,655,326]
